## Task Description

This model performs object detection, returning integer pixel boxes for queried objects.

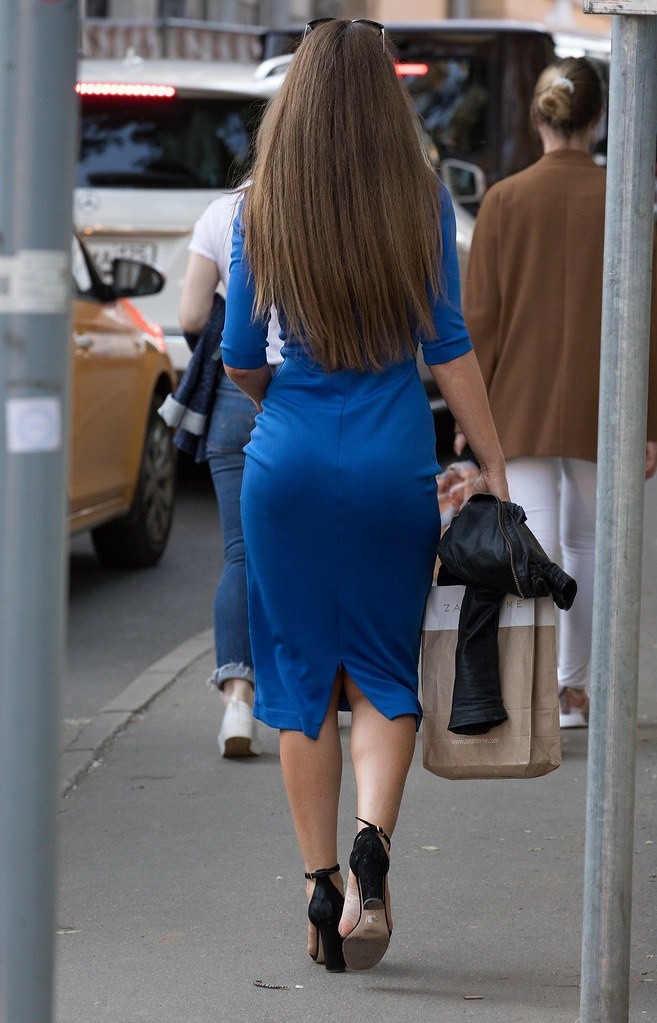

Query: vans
[282,20,557,219]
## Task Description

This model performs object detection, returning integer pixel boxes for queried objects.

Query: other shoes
[558,687,589,728]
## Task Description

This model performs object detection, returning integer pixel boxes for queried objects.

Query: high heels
[337,816,393,969]
[304,864,342,973]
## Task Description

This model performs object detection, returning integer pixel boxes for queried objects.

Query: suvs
[74,50,490,415]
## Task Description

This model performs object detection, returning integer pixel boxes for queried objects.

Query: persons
[178,55,657,765]
[220,14,515,974]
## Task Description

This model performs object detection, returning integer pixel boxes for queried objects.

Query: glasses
[303,16,386,50]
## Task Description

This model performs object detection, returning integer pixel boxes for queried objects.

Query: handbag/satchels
[423,578,560,779]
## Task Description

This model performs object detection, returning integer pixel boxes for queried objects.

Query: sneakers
[217,698,264,756]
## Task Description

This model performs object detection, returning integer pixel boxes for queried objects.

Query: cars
[68,233,180,568]
